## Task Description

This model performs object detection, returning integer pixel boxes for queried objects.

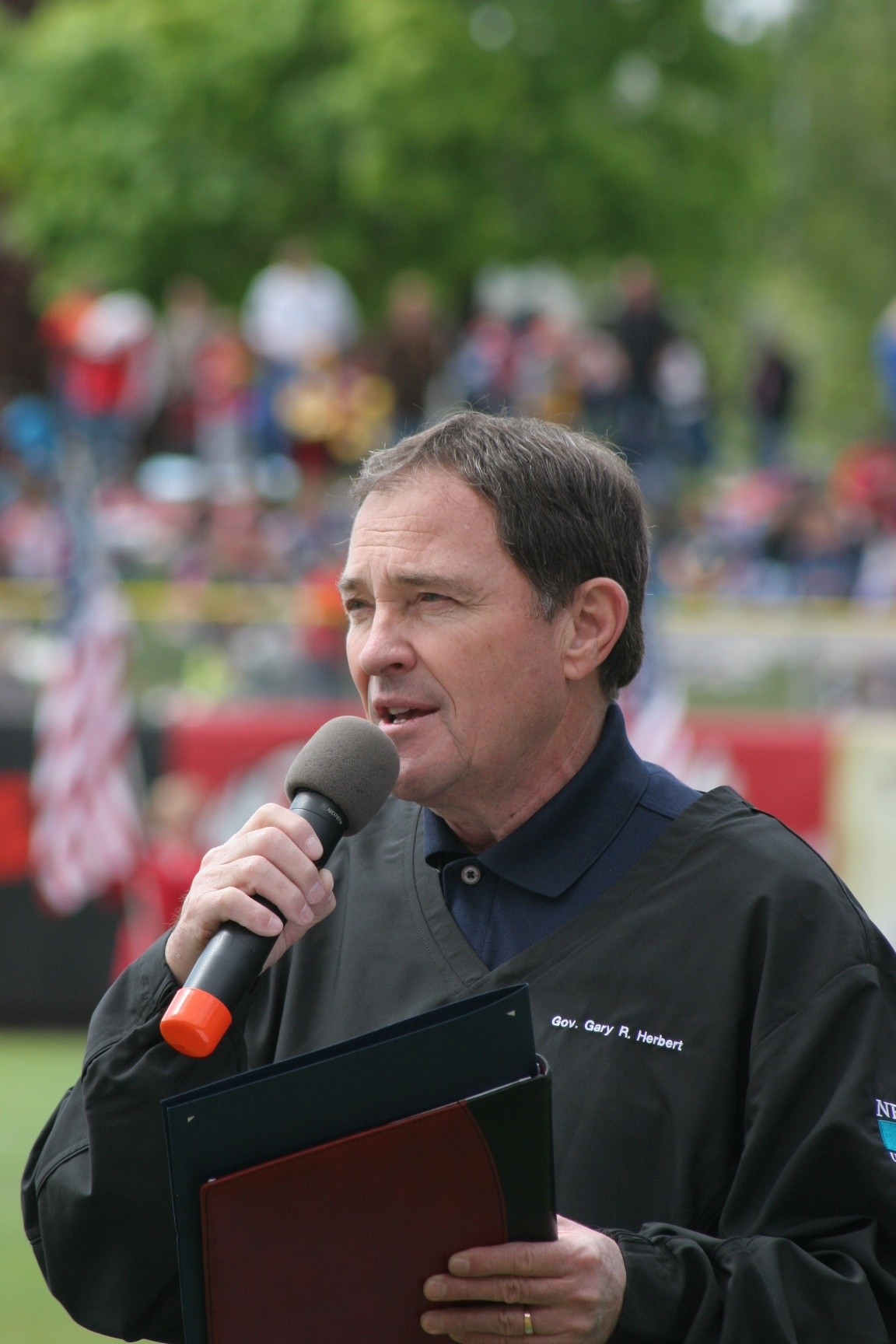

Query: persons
[0,237,896,471]
[15,412,896,1343]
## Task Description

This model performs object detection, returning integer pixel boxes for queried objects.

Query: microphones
[156,717,400,1058]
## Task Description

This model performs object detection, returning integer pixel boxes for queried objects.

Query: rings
[523,1304,534,1335]
